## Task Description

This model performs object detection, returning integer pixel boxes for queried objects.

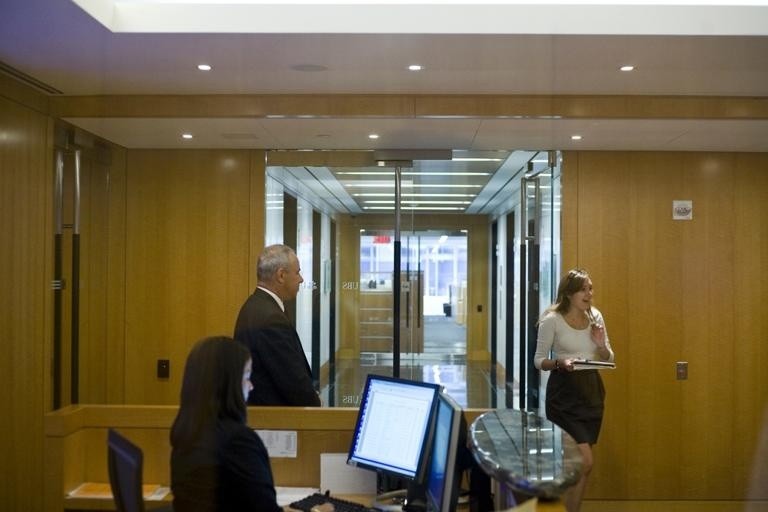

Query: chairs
[107,429,148,511]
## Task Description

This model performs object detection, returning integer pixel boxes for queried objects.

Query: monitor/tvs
[344,372,445,481]
[421,392,470,511]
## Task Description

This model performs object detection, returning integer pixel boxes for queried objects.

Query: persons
[232,244,321,405]
[533,270,614,512]
[168,335,302,512]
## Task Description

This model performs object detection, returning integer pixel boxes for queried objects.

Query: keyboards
[289,491,375,511]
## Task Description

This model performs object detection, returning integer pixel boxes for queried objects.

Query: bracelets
[554,359,560,370]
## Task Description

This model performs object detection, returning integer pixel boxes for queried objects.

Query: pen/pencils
[325,489,330,504]
